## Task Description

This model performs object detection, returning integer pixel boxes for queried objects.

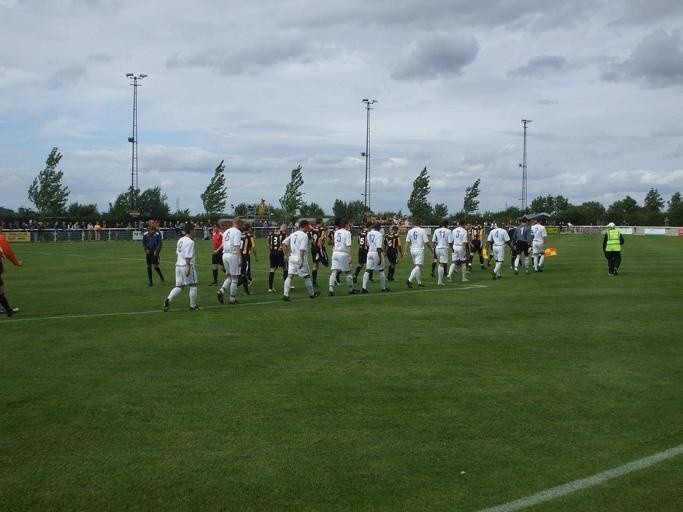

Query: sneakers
[489,263,543,279]
[190,304,200,310]
[269,283,320,301]
[328,276,394,296]
[8,307,20,316]
[164,299,169,312]
[406,275,469,289]
[207,281,255,304]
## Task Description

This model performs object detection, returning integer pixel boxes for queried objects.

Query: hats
[605,222,615,228]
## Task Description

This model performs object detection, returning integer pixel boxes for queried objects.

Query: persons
[163,223,200,311]
[601,222,624,277]
[111,217,214,241]
[252,220,277,238]
[1,219,22,317]
[209,218,257,305]
[543,219,580,232]
[267,217,487,301]
[143,225,165,287]
[484,218,548,280]
[2,219,109,242]
